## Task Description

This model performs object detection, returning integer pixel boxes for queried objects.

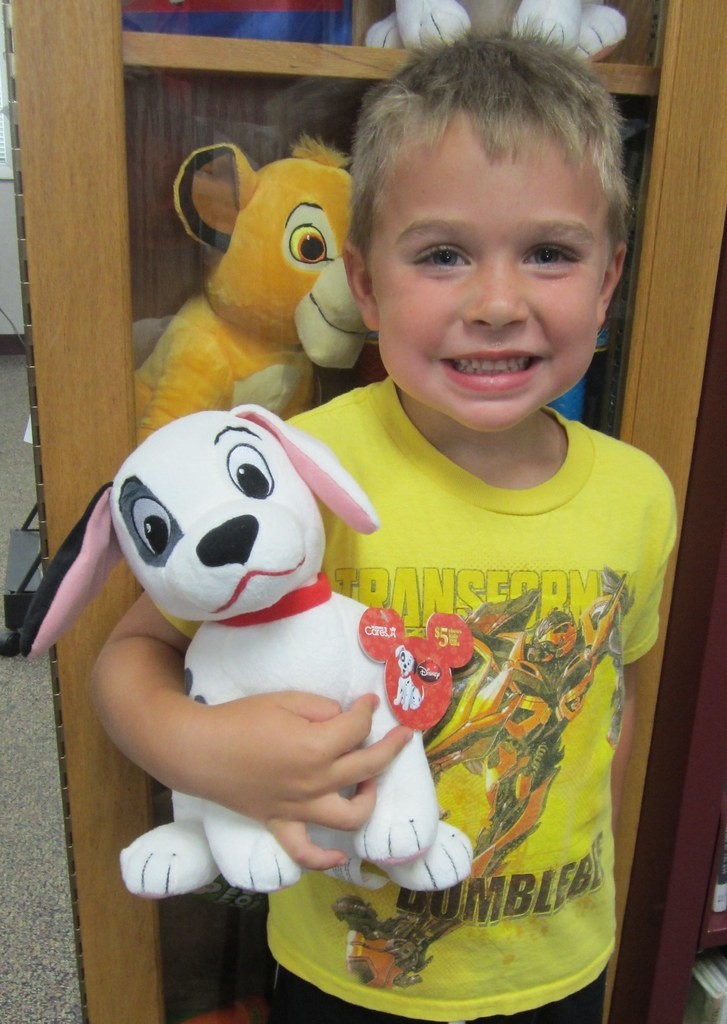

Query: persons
[84,12,683,1024]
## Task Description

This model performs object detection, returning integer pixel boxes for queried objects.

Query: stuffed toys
[21,404,478,897]
[137,133,371,445]
